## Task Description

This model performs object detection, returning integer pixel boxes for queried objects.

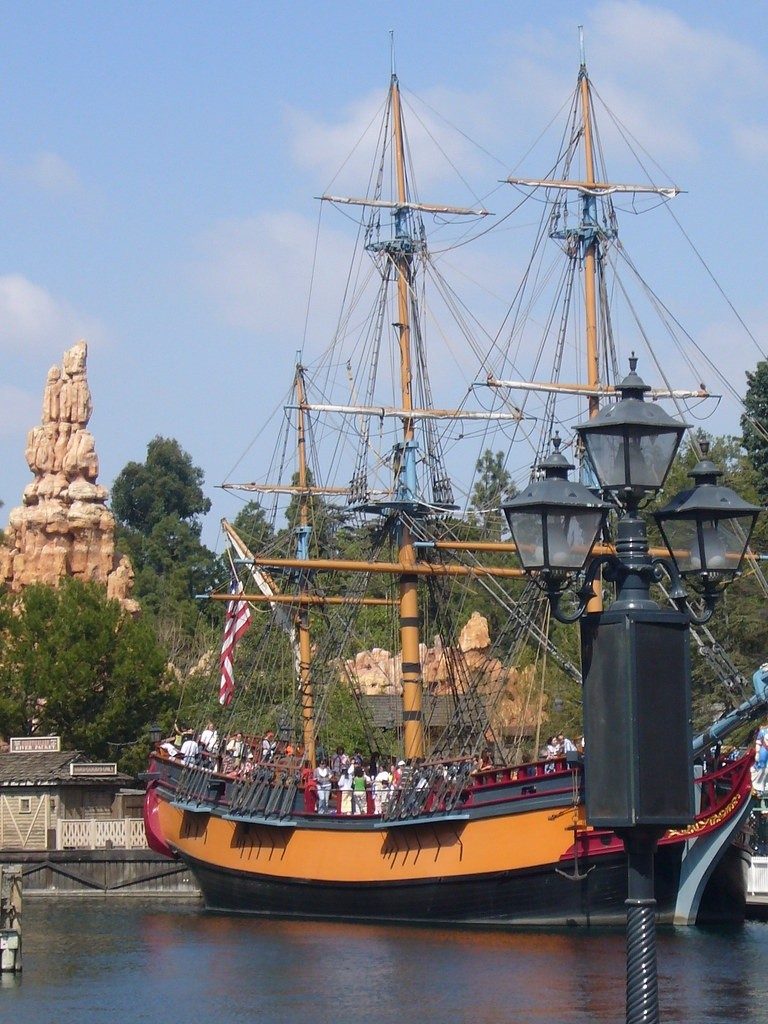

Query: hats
[342,766,347,769]
[398,760,405,765]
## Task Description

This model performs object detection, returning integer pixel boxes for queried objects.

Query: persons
[148,723,584,816]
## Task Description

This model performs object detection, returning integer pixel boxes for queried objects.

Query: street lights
[502,349,768,1024]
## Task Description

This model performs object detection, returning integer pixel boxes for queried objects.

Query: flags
[218,576,252,706]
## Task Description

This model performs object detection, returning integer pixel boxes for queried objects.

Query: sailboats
[139,59,765,929]
